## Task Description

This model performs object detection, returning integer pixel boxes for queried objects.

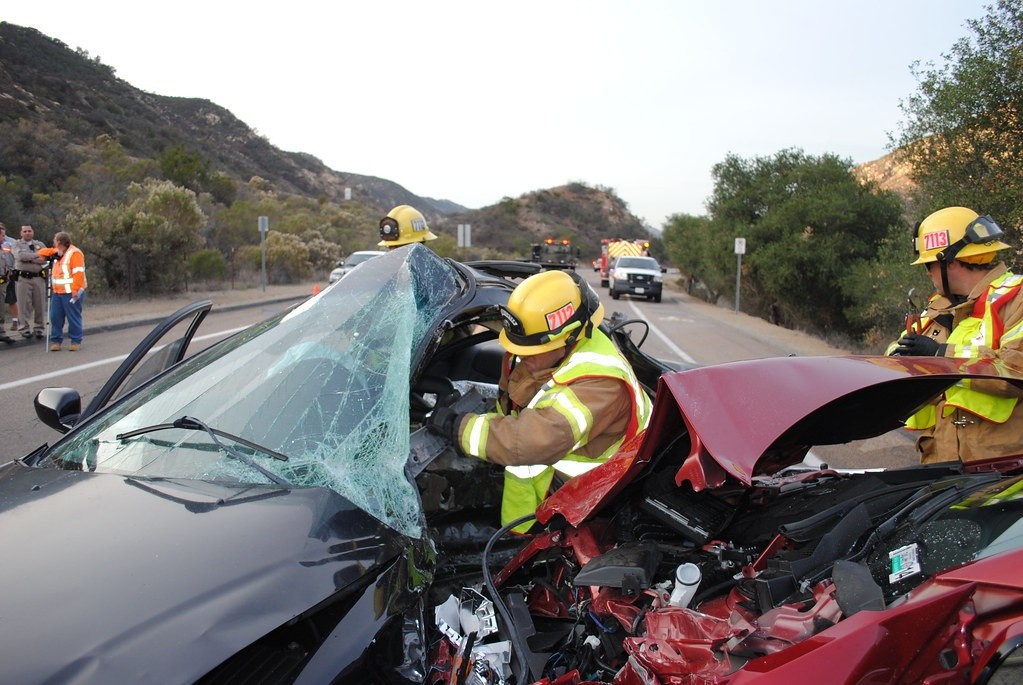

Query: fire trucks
[599,237,653,287]
[529,238,582,273]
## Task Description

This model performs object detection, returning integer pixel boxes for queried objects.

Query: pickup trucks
[608,255,667,303]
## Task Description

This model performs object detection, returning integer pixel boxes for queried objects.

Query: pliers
[906,286,923,335]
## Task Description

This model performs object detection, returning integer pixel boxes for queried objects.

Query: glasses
[967,213,1005,244]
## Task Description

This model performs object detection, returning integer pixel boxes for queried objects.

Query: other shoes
[0,336,17,343]
[69,342,80,351]
[11,320,18,331]
[23,330,31,336]
[35,331,43,337]
[51,342,59,352]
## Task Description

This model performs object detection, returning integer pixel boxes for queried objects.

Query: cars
[328,250,387,288]
[593,258,603,272]
[1,238,1023,685]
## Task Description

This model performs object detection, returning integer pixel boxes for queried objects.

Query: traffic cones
[313,284,319,296]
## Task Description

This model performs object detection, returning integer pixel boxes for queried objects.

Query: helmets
[378,204,438,247]
[910,207,1011,266]
[499,269,605,356]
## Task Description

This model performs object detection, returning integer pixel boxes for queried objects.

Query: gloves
[896,333,939,358]
[423,410,456,439]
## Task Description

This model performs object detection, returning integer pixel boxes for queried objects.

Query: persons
[10,224,48,340]
[377,204,438,252]
[422,269,653,539]
[38,232,87,351]
[884,206,1023,465]
[0,222,19,344]
